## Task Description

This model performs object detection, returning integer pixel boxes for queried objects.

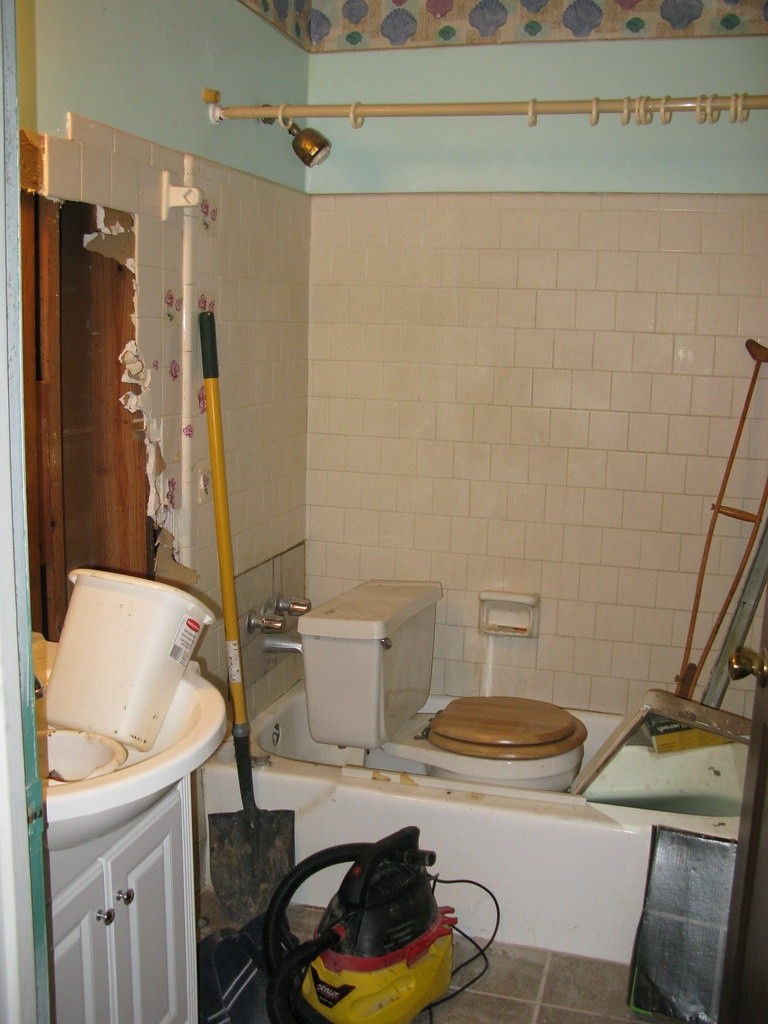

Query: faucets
[263,633,303,656]
[34,674,45,701]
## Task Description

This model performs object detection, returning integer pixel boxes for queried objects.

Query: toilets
[296,578,590,793]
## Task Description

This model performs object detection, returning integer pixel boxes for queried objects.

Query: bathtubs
[205,677,750,967]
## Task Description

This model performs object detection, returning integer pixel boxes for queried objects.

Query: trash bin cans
[40,568,217,751]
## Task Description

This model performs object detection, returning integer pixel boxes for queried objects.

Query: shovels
[199,310,295,927]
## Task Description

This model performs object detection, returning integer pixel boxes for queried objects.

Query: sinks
[47,659,229,855]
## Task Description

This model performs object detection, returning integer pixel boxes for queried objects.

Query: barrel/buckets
[38,567,216,752]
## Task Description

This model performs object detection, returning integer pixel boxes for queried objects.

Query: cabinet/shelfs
[48,773,199,1024]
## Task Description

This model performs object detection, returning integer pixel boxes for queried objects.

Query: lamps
[261,103,332,169]
